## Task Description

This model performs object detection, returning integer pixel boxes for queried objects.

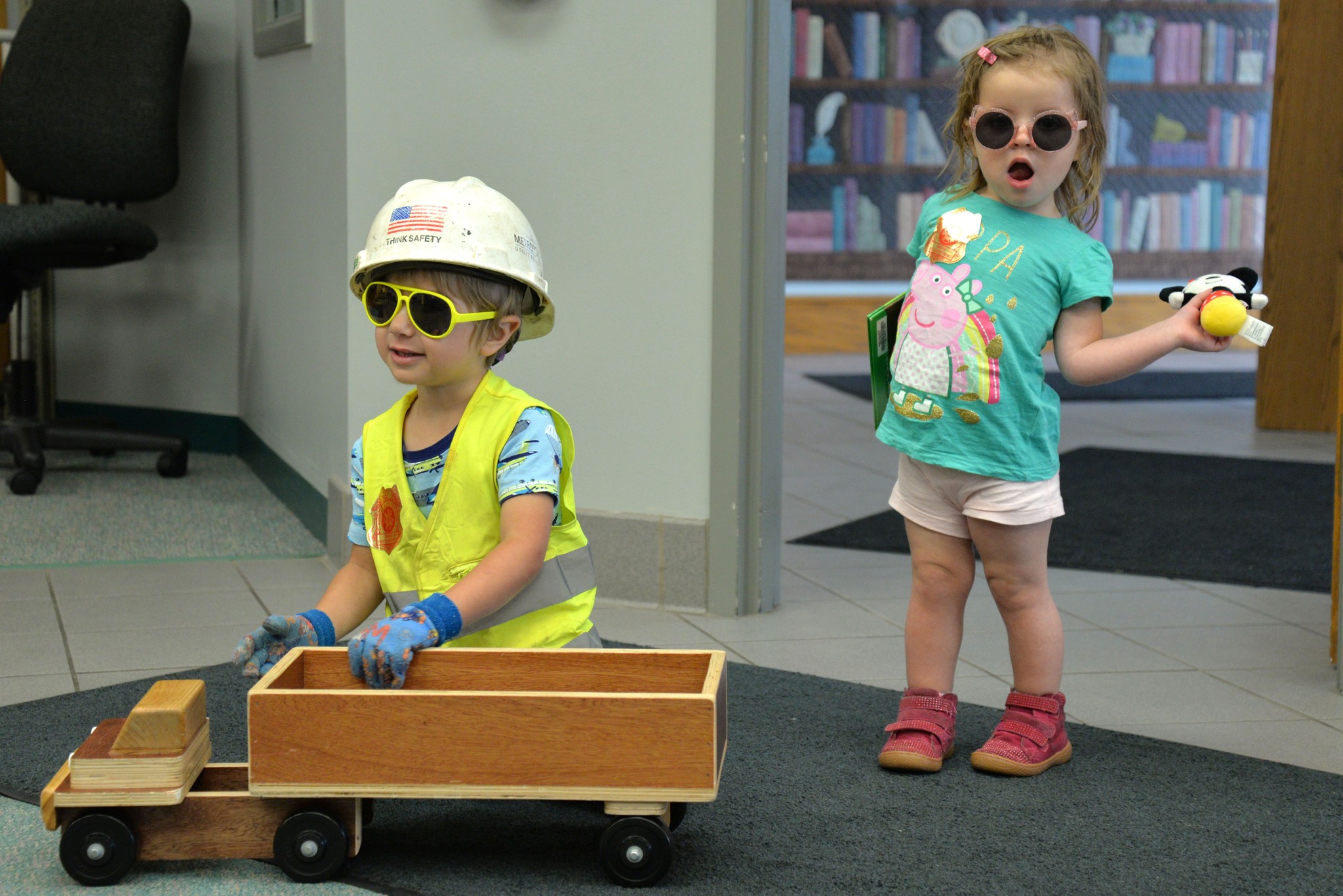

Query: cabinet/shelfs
[783,0,1282,280]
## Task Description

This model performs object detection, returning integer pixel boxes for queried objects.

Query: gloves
[233,608,335,680]
[347,594,461,689]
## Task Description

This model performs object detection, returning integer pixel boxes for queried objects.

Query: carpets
[0,638,1342,895]
[783,446,1336,593]
[802,370,1255,403]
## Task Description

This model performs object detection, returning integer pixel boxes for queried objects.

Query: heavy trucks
[41,646,736,886]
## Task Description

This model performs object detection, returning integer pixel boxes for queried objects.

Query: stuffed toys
[1159,267,1269,338]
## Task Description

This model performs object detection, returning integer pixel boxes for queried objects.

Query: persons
[874,20,1229,774]
[233,178,603,688]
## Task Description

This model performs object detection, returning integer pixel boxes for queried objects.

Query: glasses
[363,281,503,340]
[965,107,1088,152]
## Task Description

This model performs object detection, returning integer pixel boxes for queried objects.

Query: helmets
[350,177,556,341]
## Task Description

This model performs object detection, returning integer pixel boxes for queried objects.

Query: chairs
[0,0,192,498]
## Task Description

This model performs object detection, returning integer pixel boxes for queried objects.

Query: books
[1062,17,1277,252]
[787,10,948,252]
[866,293,905,431]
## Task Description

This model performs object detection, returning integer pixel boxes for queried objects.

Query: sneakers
[970,688,1071,776]
[878,688,958,772]
